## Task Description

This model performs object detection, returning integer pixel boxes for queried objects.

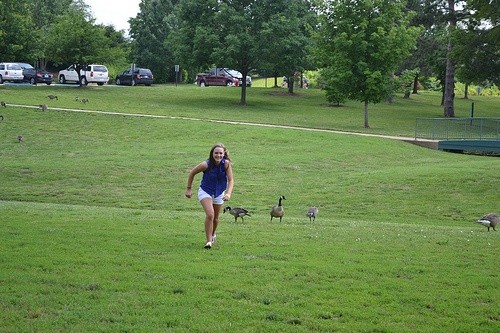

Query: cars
[195,68,253,88]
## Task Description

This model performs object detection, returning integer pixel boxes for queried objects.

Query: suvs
[57,64,110,86]
[0,62,54,86]
[116,67,155,87]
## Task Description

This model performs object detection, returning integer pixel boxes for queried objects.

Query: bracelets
[187,187,191,189]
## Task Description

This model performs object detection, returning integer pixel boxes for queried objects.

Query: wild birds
[306,207,319,222]
[0,94,91,143]
[476,213,500,232]
[270,195,286,223]
[222,206,252,224]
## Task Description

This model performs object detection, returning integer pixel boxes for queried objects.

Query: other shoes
[212,237,216,243]
[204,241,211,248]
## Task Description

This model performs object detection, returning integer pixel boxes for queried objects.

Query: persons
[302,76,308,88]
[280,76,287,88]
[186,143,234,248]
